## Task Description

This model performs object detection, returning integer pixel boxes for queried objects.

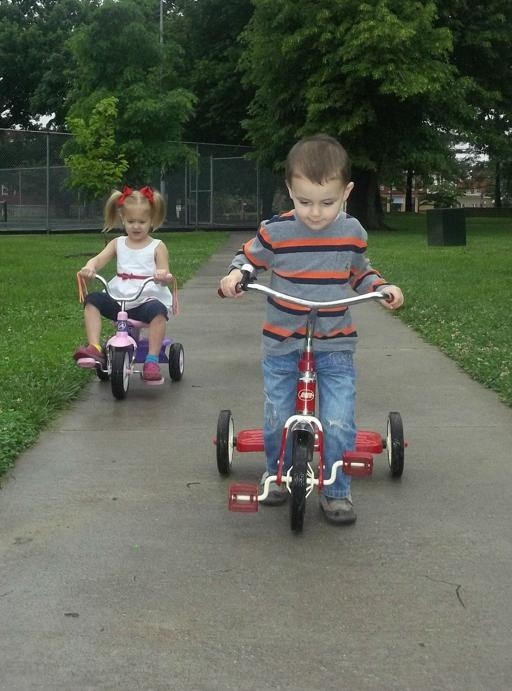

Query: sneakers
[74,344,106,363]
[259,471,288,504]
[143,362,161,380]
[319,493,357,522]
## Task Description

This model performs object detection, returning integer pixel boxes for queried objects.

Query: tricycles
[76,270,187,399]
[207,284,410,536]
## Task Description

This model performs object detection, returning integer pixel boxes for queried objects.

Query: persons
[73,189,177,383]
[216,131,408,525]
